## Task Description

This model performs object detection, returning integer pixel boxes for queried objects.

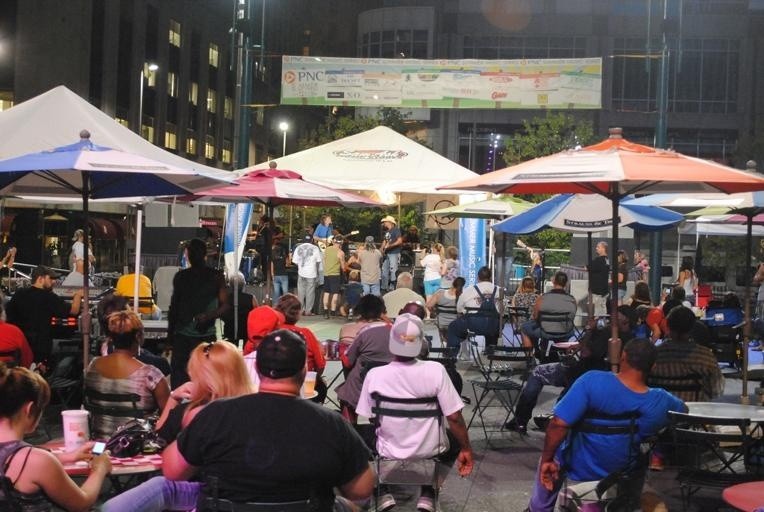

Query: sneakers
[417,497,441,512]
[377,493,396,512]
[534,413,554,428]
[506,420,526,433]
[304,309,353,320]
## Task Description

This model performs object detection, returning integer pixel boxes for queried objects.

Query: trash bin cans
[515,265,526,278]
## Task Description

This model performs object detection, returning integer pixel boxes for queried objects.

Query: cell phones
[91,440,107,455]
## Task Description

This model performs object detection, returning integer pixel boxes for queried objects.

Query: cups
[60,409,89,451]
[714,313,724,321]
[320,341,328,359]
[303,371,317,397]
[330,341,338,360]
[695,307,702,319]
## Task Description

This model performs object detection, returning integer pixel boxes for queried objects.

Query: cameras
[425,249,431,253]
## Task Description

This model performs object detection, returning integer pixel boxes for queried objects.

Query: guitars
[319,228,360,250]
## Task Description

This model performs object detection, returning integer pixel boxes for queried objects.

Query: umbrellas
[1,129,242,379]
[152,160,389,307]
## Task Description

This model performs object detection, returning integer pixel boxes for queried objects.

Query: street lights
[138,57,290,158]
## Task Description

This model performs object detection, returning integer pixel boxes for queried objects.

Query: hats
[257,329,306,379]
[381,215,396,224]
[389,313,424,357]
[32,265,62,278]
[304,234,312,242]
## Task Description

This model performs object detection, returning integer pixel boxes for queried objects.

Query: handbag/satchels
[104,419,147,457]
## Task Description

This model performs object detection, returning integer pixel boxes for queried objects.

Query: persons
[0,367,205,511]
[253,214,459,319]
[340,273,474,512]
[168,238,227,389]
[525,339,688,512]
[1,216,16,269]
[426,241,764,469]
[91,260,324,439]
[161,329,375,511]
[1,264,85,369]
[156,340,260,442]
[63,229,97,288]
